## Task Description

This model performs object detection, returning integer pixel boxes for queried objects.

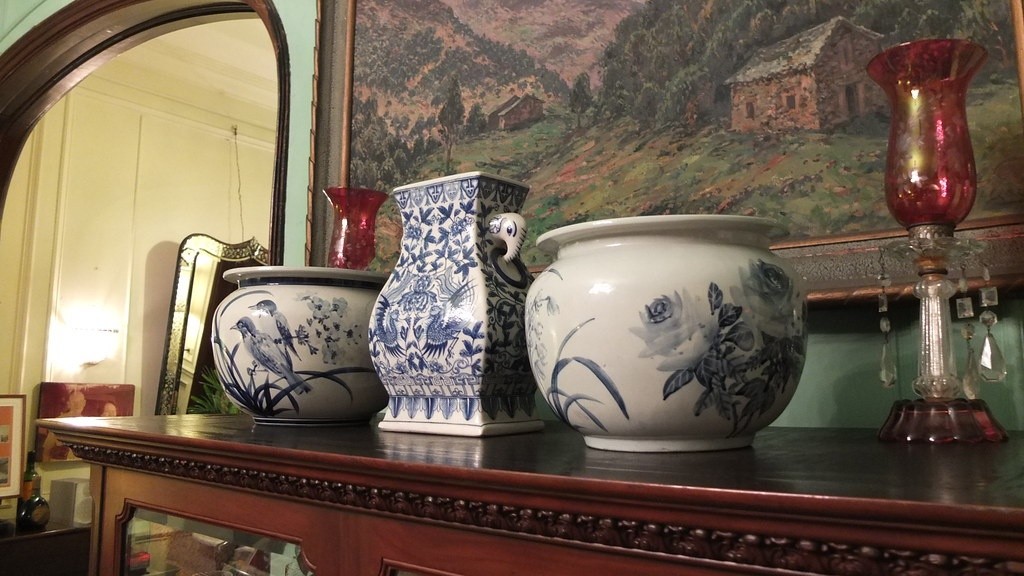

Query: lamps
[863,36,1009,448]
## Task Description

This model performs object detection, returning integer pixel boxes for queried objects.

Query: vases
[521,213,808,454]
[366,171,548,439]
[209,264,391,428]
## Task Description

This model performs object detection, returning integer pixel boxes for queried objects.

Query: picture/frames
[0,393,27,501]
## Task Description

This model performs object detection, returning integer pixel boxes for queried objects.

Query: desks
[33,412,1024,576]
